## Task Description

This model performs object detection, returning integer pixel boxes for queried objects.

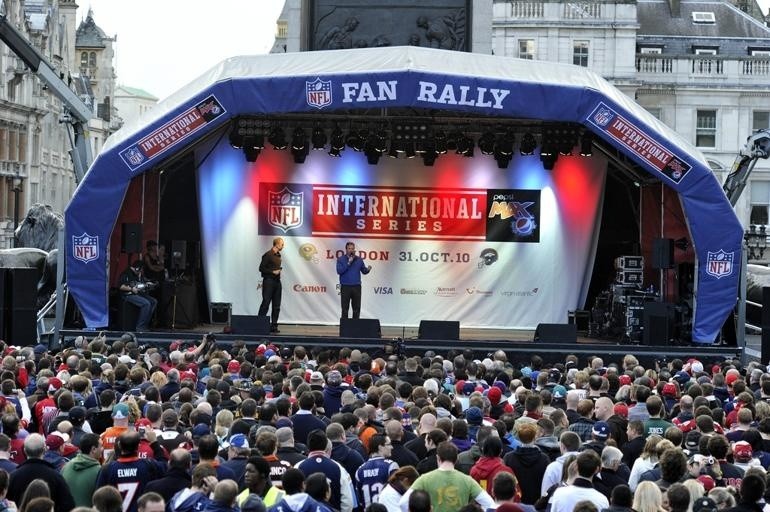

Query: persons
[144,240,163,332]
[337,242,371,319]
[2,333,770,512]
[118,260,158,333]
[258,237,284,333]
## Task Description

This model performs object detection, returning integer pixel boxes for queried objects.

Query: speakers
[419,320,459,340]
[171,240,194,270]
[231,315,270,335]
[653,238,674,269]
[121,223,143,251]
[161,283,200,327]
[674,263,694,320]
[339,318,381,338]
[535,324,577,344]
[644,302,676,346]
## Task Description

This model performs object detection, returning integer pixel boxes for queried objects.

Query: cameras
[705,458,714,466]
[139,427,145,436]
[207,332,216,343]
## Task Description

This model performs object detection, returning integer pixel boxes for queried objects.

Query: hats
[109,401,129,422]
[549,381,569,403]
[414,365,522,413]
[172,340,344,393]
[42,429,75,463]
[587,418,612,442]
[3,335,69,396]
[729,433,755,461]
[145,239,158,248]
[663,380,680,399]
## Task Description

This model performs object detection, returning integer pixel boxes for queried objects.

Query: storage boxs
[616,256,643,270]
[616,271,644,285]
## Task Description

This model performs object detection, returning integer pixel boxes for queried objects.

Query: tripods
[163,270,192,330]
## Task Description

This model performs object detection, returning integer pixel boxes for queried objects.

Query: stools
[568,308,593,336]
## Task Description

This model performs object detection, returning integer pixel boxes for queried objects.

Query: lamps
[433,133,448,155]
[559,142,573,156]
[388,140,405,159]
[497,140,514,158]
[394,123,427,143]
[292,127,306,150]
[405,142,427,159]
[271,133,288,151]
[479,136,494,155]
[580,141,593,157]
[456,137,470,155]
[519,132,536,156]
[352,129,368,153]
[375,129,388,153]
[540,140,553,157]
[236,117,273,138]
[311,129,325,151]
[228,129,266,163]
[327,128,345,158]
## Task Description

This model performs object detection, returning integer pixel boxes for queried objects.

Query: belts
[263,277,281,281]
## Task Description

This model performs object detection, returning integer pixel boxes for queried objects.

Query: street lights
[8,172,25,248]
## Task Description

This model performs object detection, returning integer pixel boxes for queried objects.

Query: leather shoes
[270,327,281,333]
